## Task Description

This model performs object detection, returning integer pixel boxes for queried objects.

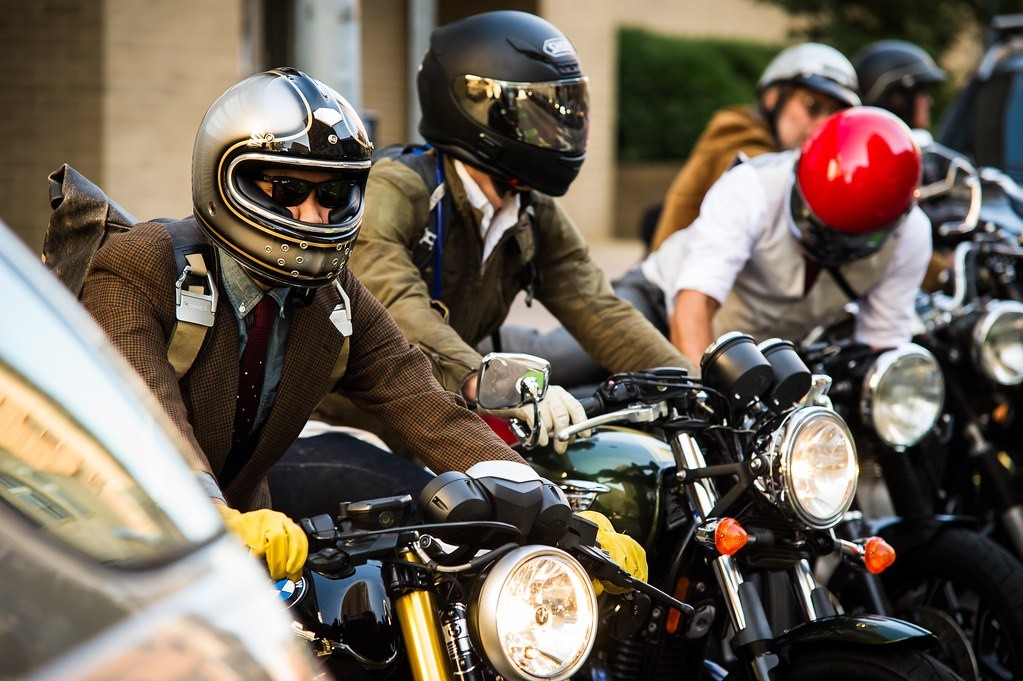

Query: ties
[221,294,279,484]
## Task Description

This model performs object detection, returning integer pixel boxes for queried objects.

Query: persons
[345,8,691,455]
[474,37,1023,380]
[77,9,648,594]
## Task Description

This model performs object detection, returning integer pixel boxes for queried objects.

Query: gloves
[567,510,649,596]
[211,500,308,582]
[475,384,592,454]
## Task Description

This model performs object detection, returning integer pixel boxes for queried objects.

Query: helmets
[847,39,948,107]
[792,106,920,263]
[191,66,375,289]
[417,9,588,197]
[755,42,862,108]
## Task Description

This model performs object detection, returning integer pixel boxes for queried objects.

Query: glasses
[804,96,840,121]
[249,173,358,209]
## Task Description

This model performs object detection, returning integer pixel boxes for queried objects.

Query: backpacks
[40,164,350,415]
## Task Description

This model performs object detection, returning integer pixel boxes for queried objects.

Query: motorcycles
[1,129,1022,681]
